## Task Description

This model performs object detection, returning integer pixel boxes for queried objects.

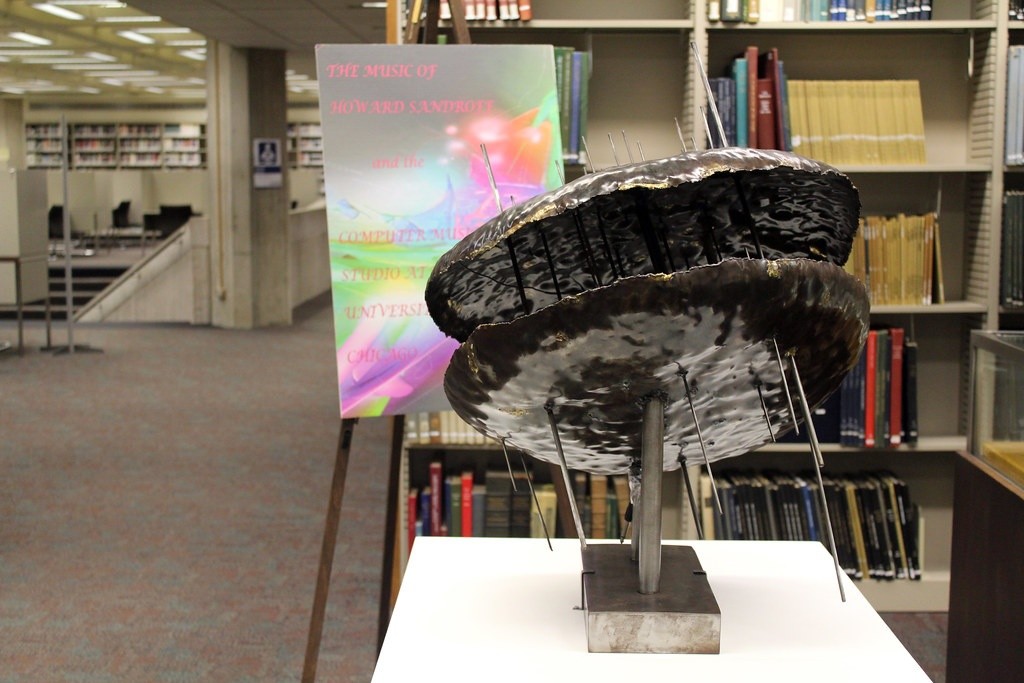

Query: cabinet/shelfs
[388,0,1024,610]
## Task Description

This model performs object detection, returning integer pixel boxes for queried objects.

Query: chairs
[160,204,200,243]
[139,212,185,256]
[49,205,84,260]
[106,201,141,255]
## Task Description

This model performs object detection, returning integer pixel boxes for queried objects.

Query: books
[397,0,1024,584]
[287,124,323,165]
[25,122,202,170]
